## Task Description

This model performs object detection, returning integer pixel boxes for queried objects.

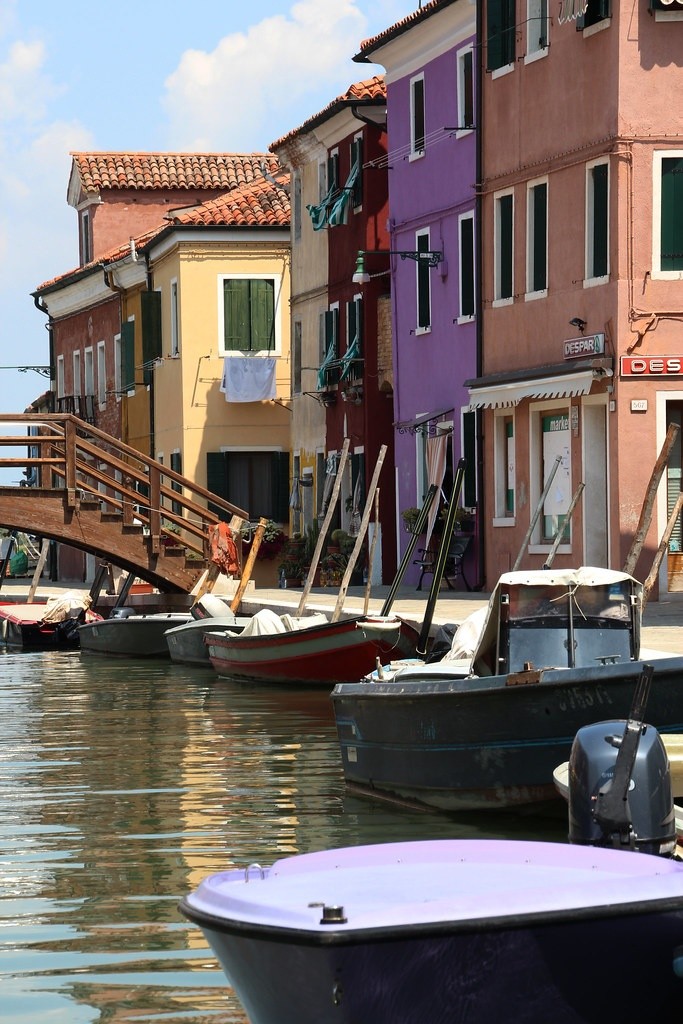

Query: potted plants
[440,507,474,535]
[279,531,365,589]
[161,522,182,546]
[401,507,420,530]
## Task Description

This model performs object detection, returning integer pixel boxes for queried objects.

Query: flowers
[245,520,280,542]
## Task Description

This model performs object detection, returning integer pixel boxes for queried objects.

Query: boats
[329,567,683,822]
[1,594,105,653]
[76,605,194,659]
[178,721,682,1023]
[161,593,254,669]
[204,606,432,689]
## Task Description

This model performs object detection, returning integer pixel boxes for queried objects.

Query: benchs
[414,535,475,591]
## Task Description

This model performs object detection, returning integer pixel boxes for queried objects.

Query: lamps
[352,250,443,285]
[569,318,584,330]
